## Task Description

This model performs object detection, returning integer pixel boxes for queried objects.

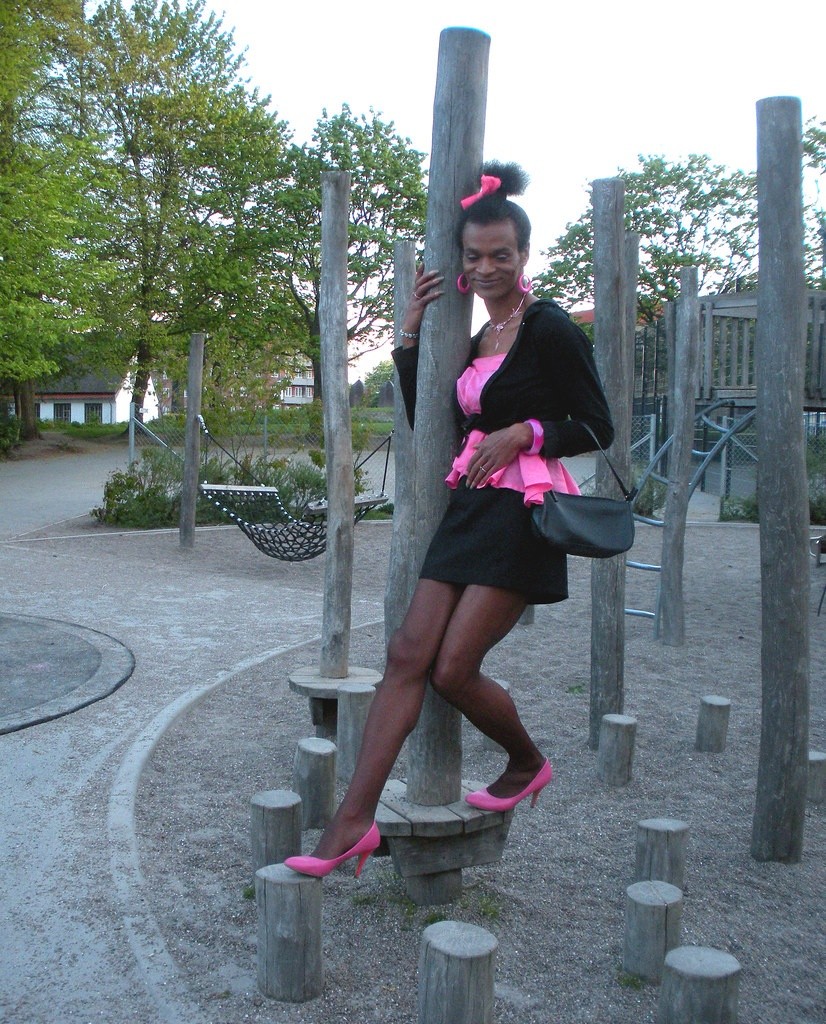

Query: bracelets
[399,329,418,339]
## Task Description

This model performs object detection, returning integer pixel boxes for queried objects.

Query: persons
[279,196,615,879]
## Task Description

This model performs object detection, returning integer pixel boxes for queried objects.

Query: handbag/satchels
[529,419,637,559]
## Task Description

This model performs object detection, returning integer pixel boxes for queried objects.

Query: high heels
[465,757,553,811]
[284,821,381,878]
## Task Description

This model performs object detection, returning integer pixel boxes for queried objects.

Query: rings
[413,292,421,298]
[480,466,487,473]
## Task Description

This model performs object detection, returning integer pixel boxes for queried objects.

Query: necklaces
[488,292,526,351]
